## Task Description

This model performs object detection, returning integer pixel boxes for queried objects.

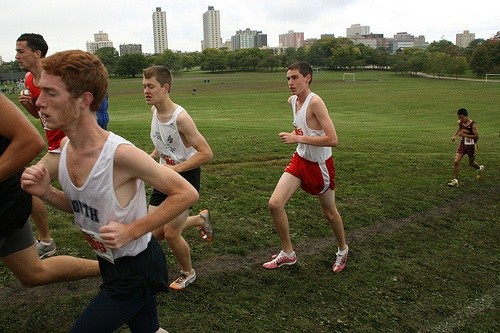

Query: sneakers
[169,269,196,290]
[35,238,56,259]
[476,165,484,179]
[332,245,349,272]
[263,250,297,269]
[448,179,458,188]
[199,209,214,243]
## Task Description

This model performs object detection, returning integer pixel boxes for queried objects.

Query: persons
[21,50,199,333]
[448,107,485,187]
[0,92,102,287]
[262,61,348,273]
[141,64,213,289]
[96,89,109,129]
[16,33,69,259]
